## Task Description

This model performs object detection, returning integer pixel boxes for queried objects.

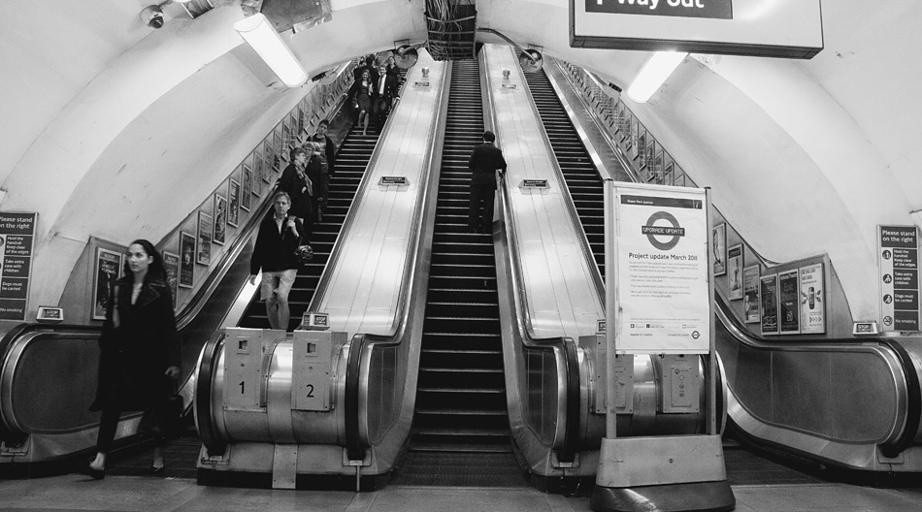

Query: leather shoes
[150,465,164,475]
[82,464,104,480]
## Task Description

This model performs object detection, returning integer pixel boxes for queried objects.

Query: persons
[279,147,315,245]
[342,54,407,135]
[80,239,181,479]
[306,119,335,213]
[469,131,507,234]
[301,142,321,228]
[250,191,310,331]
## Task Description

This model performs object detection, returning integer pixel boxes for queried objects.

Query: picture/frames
[712,222,826,336]
[263,105,305,185]
[90,216,197,327]
[197,150,263,268]
[603,95,632,151]
[631,121,685,186]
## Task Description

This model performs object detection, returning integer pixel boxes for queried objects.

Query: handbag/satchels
[295,244,313,263]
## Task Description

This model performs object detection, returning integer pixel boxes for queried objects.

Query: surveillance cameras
[141,5,164,29]
